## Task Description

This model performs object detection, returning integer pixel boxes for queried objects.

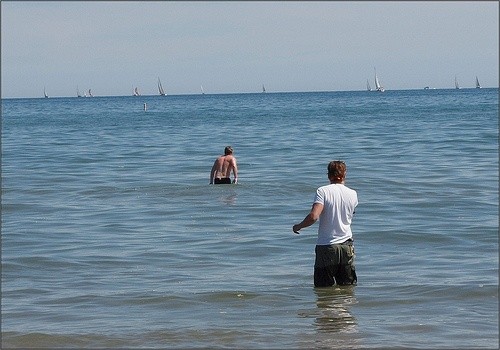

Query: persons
[293,161,357,288]
[209,145,238,185]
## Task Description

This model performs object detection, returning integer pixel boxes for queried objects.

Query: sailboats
[366,79,373,92]
[454,75,462,90]
[262,85,268,94]
[200,84,206,95]
[43,88,49,99]
[156,77,168,97]
[375,74,385,92]
[76,85,95,98]
[133,87,142,97]
[476,76,481,89]
